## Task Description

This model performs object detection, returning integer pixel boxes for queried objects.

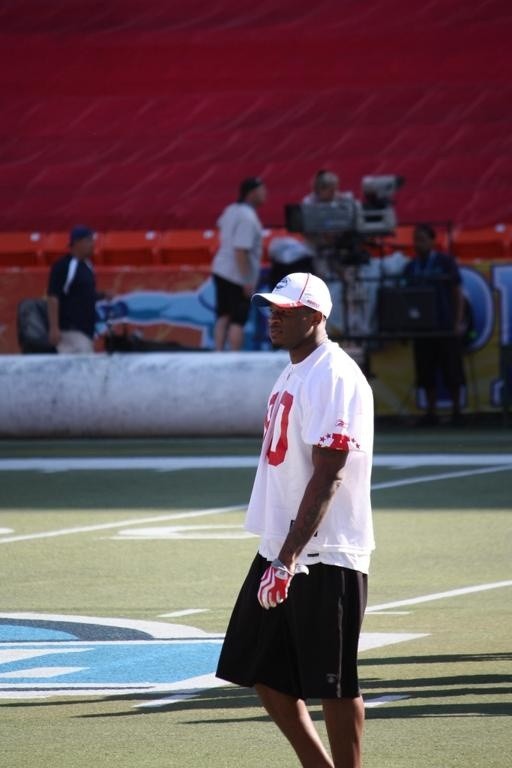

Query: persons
[208,172,272,352]
[398,223,472,428]
[297,168,366,235]
[210,268,380,767]
[43,223,121,354]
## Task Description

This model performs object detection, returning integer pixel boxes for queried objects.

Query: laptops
[374,287,433,332]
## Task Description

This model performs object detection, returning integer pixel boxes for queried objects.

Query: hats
[250,272,332,320]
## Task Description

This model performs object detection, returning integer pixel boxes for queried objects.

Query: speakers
[16,296,51,352]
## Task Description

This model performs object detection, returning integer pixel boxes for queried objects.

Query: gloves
[257,558,310,610]
